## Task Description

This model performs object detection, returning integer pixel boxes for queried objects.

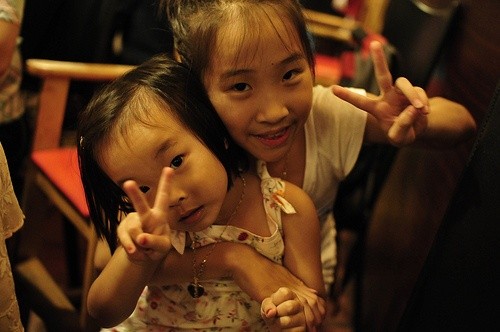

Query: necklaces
[187,173,246,299]
[267,153,290,180]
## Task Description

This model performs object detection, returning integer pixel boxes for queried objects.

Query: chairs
[23,59,138,332]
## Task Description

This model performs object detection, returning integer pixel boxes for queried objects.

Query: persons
[94,0,478,332]
[77,57,326,332]
[0,0,171,332]
